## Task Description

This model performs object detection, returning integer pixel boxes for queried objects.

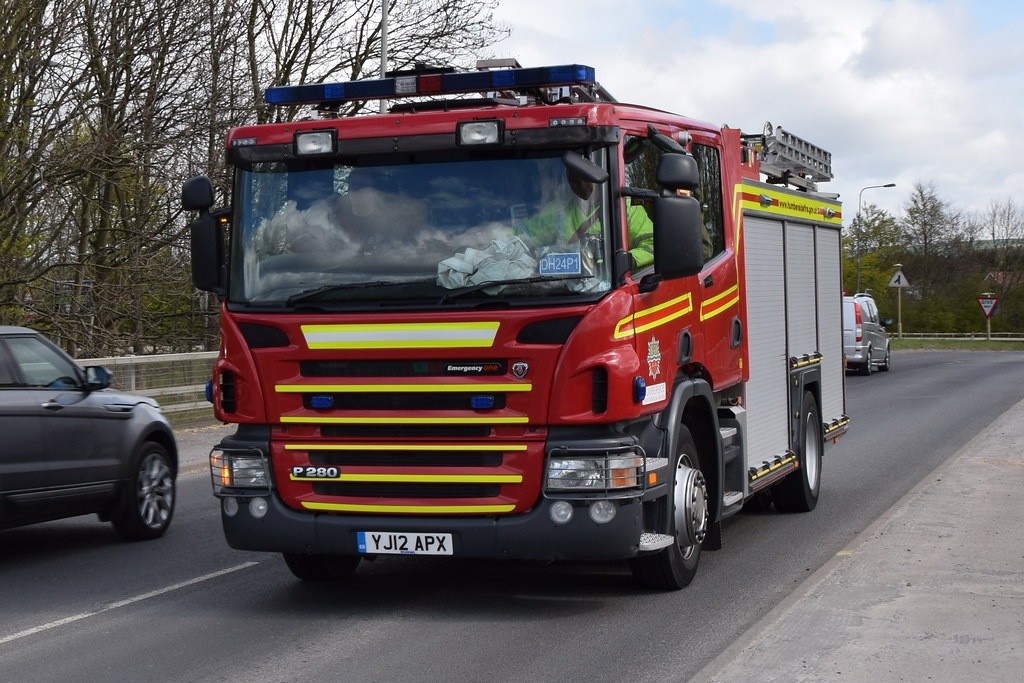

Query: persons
[280,169,406,256]
[513,173,654,271]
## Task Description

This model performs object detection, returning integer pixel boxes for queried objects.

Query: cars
[0,324,179,542]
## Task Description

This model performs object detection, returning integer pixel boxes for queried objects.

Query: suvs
[843,290,895,376]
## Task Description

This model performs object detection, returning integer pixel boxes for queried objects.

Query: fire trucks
[180,57,854,591]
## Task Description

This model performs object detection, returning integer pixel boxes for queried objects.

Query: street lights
[854,183,898,294]
[892,263,904,341]
